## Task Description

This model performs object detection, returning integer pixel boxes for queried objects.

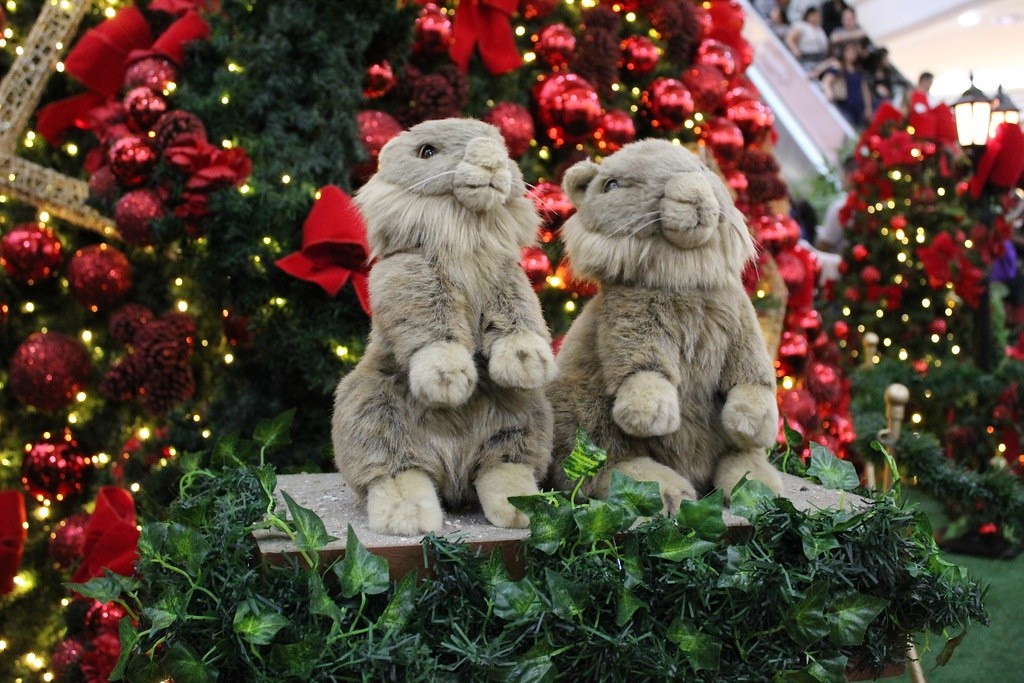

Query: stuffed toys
[330,117,559,534]
[541,138,788,516]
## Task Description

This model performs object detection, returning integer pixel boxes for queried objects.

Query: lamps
[949,72,1018,150]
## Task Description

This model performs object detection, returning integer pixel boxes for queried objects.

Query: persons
[751,0,935,139]
[786,153,855,289]
